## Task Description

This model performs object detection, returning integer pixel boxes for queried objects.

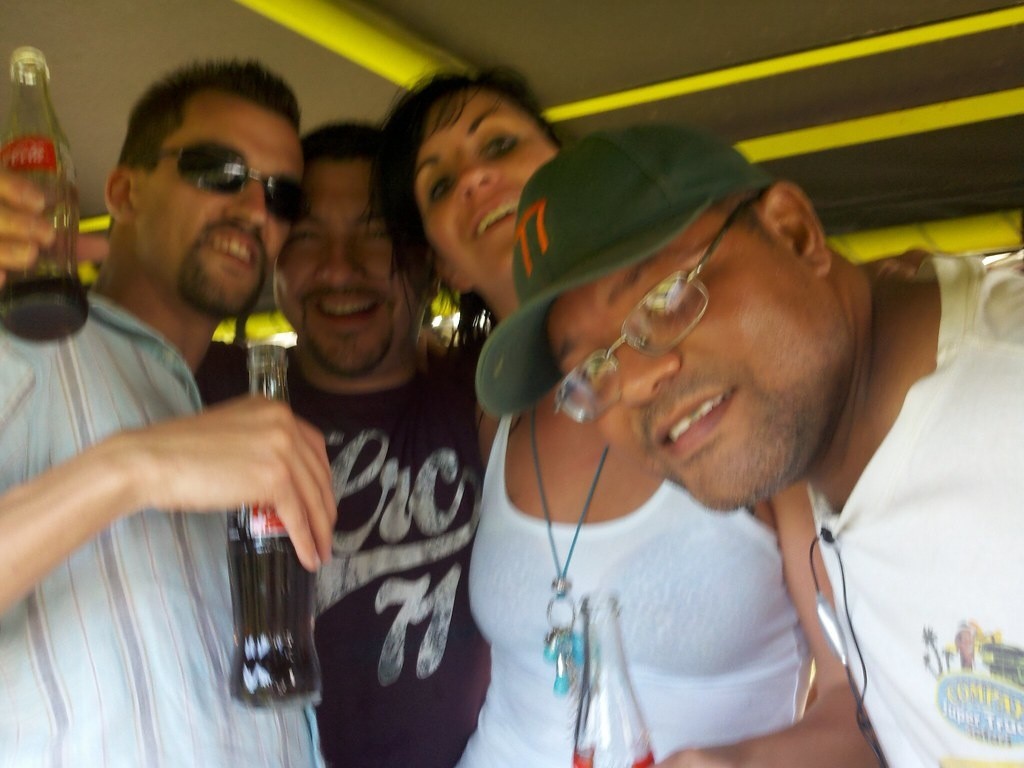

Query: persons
[0,58,338,768]
[0,120,500,768]
[372,71,885,768]
[473,122,1024,768]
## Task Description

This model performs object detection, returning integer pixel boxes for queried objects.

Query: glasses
[544,189,768,425]
[124,141,312,225]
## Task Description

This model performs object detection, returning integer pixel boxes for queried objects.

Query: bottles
[570,593,655,768]
[227,341,321,709]
[0,44,92,342]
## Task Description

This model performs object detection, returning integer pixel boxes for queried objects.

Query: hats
[475,123,775,421]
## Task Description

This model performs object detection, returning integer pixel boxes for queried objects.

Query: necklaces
[529,401,610,698]
